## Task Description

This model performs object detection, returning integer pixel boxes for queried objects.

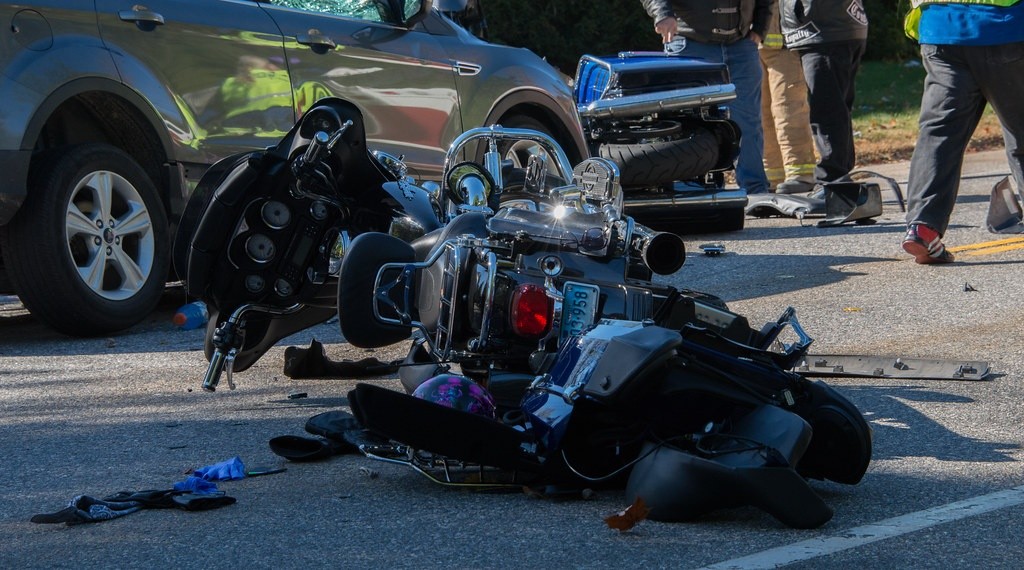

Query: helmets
[411,373,496,418]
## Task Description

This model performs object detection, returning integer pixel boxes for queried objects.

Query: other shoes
[776,179,816,194]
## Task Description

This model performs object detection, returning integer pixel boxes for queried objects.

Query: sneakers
[901,223,954,264]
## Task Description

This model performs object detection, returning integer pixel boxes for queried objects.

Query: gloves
[193,455,245,483]
[303,411,409,457]
[131,489,236,511]
[269,435,354,463]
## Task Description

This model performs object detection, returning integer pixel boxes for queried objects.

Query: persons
[778,0,868,213]
[199,54,334,147]
[639,0,776,194]
[758,0,817,193]
[903,0,1024,263]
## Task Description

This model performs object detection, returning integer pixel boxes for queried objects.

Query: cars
[1,1,600,347]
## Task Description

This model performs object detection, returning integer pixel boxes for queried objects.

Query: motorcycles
[569,48,746,187]
[169,96,872,533]
